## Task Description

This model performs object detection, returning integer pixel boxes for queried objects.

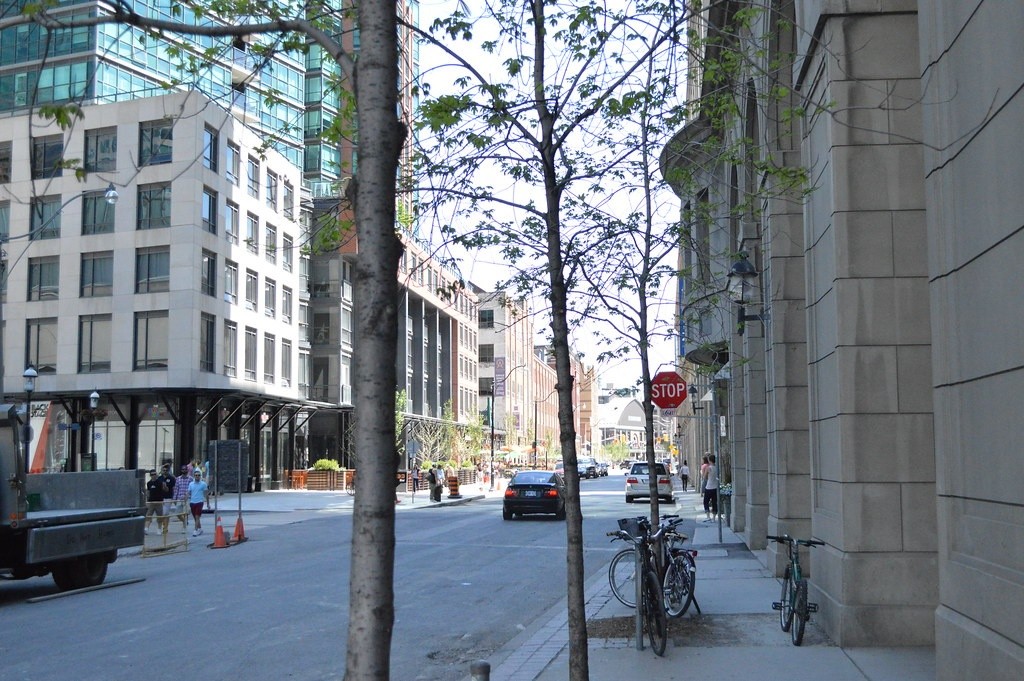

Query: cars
[555,458,610,479]
[619,459,674,503]
[502,470,566,521]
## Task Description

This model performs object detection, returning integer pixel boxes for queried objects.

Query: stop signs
[651,371,687,410]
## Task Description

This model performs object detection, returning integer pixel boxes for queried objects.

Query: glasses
[182,469,187,470]
[150,473,156,476]
[195,475,200,476]
[163,470,168,471]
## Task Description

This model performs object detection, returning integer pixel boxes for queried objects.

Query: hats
[162,464,170,468]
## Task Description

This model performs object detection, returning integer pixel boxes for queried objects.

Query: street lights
[489,364,527,492]
[22,362,39,473]
[90,386,100,472]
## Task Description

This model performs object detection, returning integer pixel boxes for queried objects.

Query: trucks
[0,405,148,592]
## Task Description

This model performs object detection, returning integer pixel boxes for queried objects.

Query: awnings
[0,387,337,416]
[395,410,485,434]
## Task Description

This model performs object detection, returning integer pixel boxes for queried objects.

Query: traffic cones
[230,517,248,541]
[497,481,501,490]
[210,516,230,549]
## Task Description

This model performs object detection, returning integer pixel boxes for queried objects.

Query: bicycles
[606,514,698,656]
[766,534,825,646]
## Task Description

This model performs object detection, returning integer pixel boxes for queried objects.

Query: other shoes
[193,528,203,536]
[144,528,149,535]
[157,527,162,535]
[182,521,189,534]
[703,518,715,523]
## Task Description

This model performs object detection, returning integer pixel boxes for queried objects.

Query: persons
[118,457,210,537]
[475,460,503,490]
[411,463,420,492]
[680,460,689,492]
[700,455,719,522]
[427,464,444,502]
[394,478,401,504]
